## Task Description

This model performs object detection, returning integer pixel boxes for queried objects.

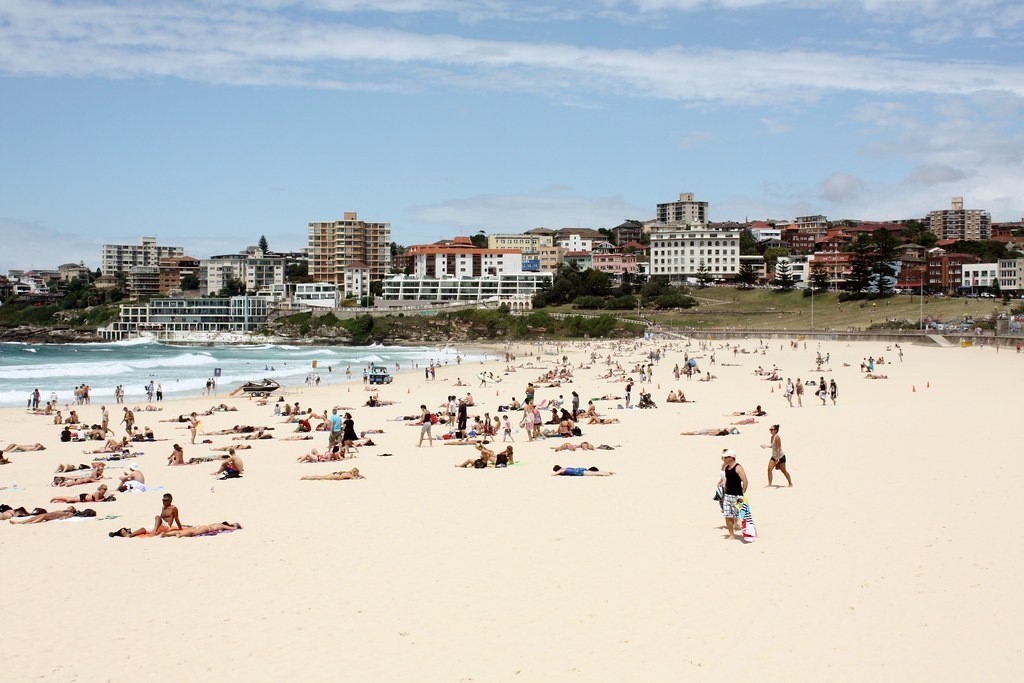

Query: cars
[893,288,1024,300]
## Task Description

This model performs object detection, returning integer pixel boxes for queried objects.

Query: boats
[242,379,280,396]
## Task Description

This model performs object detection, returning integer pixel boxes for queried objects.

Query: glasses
[769,429,775,431]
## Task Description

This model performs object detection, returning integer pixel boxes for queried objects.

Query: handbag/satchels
[430,413,438,426]
[522,412,534,424]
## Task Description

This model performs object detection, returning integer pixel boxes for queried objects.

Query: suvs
[368,366,393,385]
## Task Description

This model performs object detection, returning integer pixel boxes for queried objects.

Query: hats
[721,449,736,459]
[630,382,634,385]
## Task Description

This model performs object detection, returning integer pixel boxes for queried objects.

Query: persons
[731,406,767,417]
[168,444,183,466]
[116,463,144,491]
[975,327,983,335]
[552,465,615,476]
[1016,343,1021,353]
[416,404,432,447]
[680,427,739,436]
[301,468,366,481]
[0,362,618,487]
[761,424,793,487]
[993,327,998,336]
[10,506,76,524]
[0,507,30,521]
[121,493,238,539]
[730,418,754,425]
[717,449,748,540]
[1010,327,1020,334]
[430,300,904,409]
[50,484,113,503]
